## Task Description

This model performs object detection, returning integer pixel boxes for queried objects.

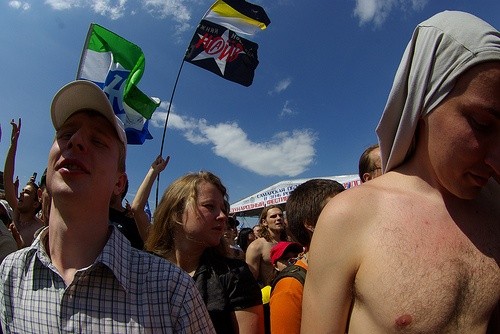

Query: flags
[183,0,271,87]
[76,24,161,145]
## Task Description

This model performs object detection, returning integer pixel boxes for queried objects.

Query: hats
[270,241,303,265]
[50,80,127,155]
[227,217,240,227]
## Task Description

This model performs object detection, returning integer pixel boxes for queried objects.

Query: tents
[227,174,363,217]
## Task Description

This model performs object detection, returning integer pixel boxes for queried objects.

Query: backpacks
[261,264,307,334]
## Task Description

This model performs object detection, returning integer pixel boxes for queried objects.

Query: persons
[108,153,170,253]
[270,179,347,334]
[222,205,307,334]
[144,170,265,334]
[0,80,238,334]
[359,144,384,183]
[0,117,53,264]
[299,10,500,334]
